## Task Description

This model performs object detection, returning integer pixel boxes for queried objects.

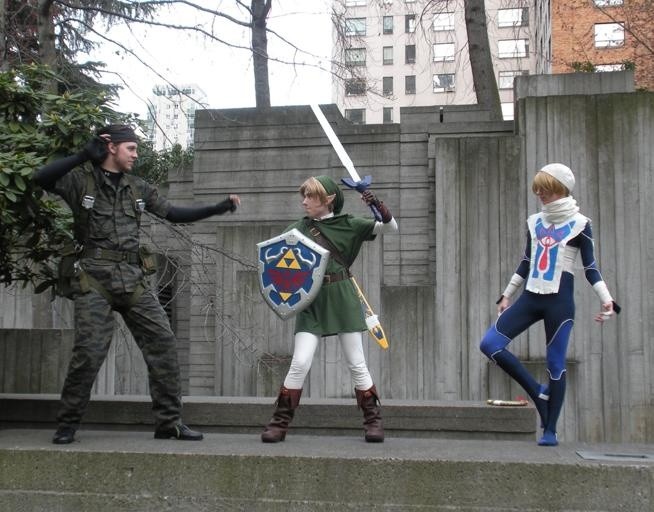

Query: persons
[28,121,243,445]
[476,162,624,448]
[258,172,399,446]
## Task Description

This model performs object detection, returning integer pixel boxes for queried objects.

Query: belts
[83,247,139,265]
[322,268,349,284]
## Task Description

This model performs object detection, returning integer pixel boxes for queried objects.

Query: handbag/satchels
[55,239,88,299]
[139,244,158,277]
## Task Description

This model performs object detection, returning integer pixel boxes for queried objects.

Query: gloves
[362,190,391,224]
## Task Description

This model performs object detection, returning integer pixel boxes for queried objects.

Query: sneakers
[539,385,551,427]
[52,426,74,443]
[153,424,203,440]
[538,432,558,446]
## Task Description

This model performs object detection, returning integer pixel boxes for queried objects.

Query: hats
[95,124,136,145]
[540,162,575,195]
[315,174,344,215]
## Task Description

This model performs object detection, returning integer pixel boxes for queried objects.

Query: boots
[353,385,384,443]
[260,384,303,443]
